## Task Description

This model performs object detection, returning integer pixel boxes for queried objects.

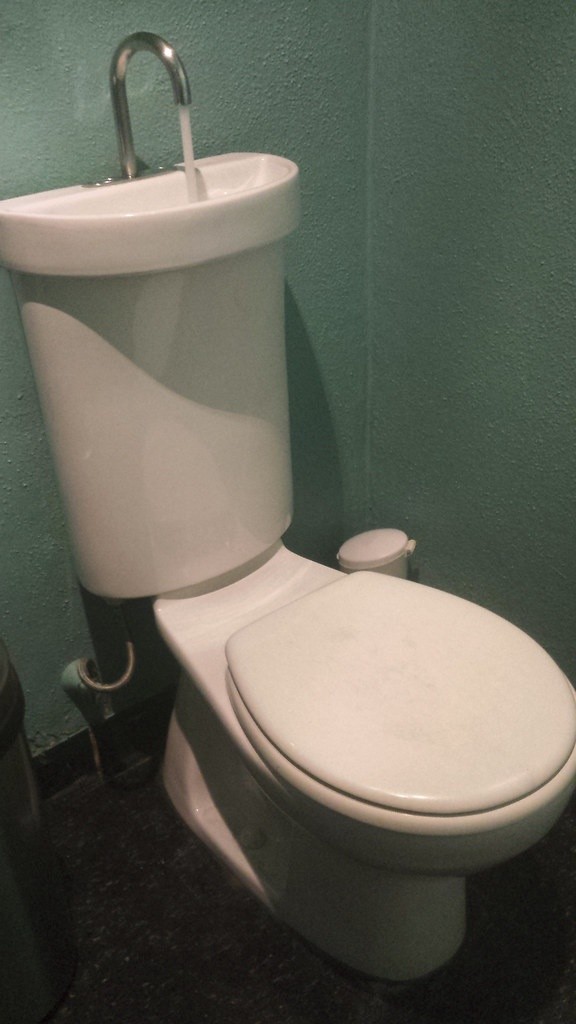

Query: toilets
[153,537,576,984]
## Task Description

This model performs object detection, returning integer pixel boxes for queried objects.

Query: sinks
[0,150,303,278]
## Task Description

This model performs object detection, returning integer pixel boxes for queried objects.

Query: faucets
[108,29,193,179]
